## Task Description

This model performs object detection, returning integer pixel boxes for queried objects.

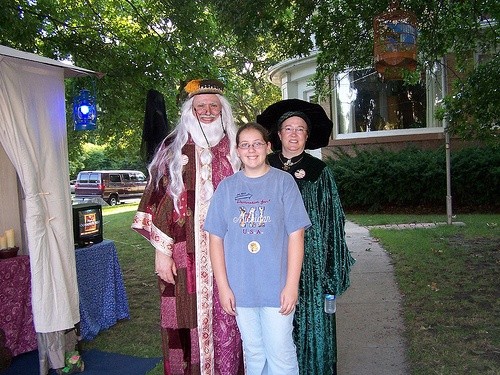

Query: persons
[256,98,356,375]
[131,78,244,375]
[203,122,312,375]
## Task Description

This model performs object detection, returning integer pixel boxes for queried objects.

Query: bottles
[324,293,336,314]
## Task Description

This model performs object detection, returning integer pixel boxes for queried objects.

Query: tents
[0,45,103,375]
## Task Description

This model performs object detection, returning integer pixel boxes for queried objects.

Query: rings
[154,272,158,274]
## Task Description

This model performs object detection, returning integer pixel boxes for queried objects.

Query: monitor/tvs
[72,203,103,249]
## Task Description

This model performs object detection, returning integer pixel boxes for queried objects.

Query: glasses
[238,142,266,148]
[279,126,308,135]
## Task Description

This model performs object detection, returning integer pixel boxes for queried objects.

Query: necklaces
[279,155,303,170]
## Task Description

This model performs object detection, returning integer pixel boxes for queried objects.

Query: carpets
[0,347,164,375]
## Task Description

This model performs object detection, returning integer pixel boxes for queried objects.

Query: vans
[74,170,148,206]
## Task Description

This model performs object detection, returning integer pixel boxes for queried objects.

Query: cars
[70,179,77,194]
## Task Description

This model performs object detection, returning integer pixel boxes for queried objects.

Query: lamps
[67,71,99,133]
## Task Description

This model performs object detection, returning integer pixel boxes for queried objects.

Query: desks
[0,255,38,372]
[72,240,131,342]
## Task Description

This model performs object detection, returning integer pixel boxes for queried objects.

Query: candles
[0,228,16,250]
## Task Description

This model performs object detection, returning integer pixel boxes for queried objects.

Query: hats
[183,78,224,98]
[256,98,333,150]
